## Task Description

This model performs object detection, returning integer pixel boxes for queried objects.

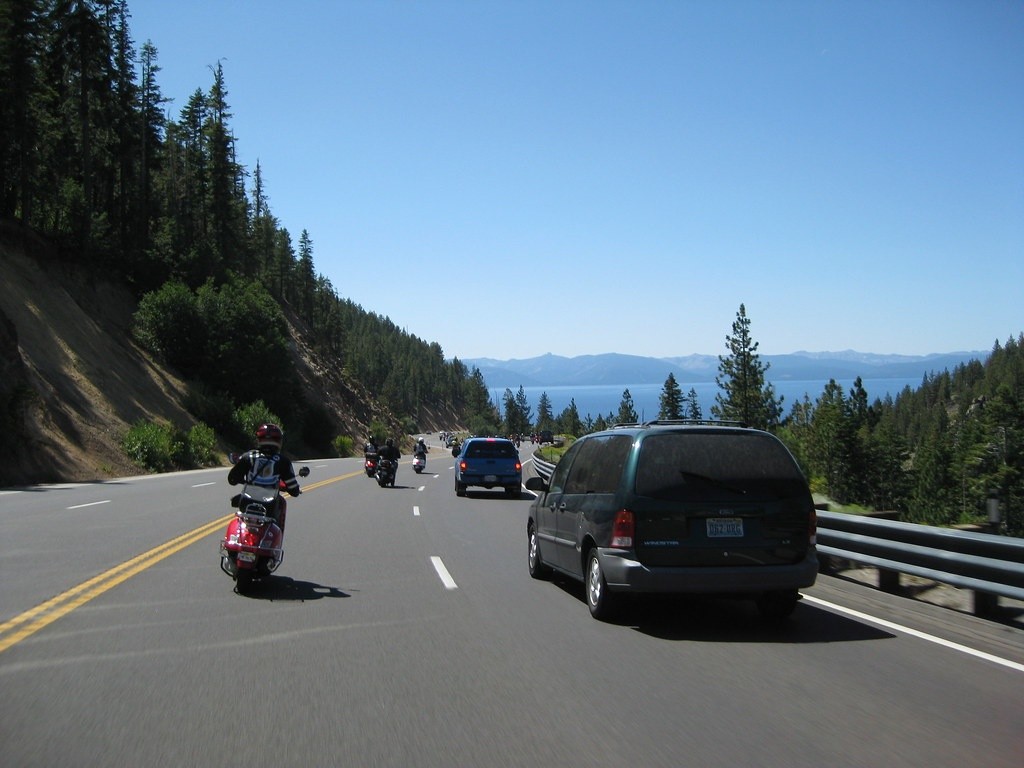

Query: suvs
[538,430,555,445]
[454,438,522,500]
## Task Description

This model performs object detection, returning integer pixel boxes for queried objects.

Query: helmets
[369,436,374,442]
[454,437,457,440]
[255,424,283,450]
[385,438,393,446]
[418,437,424,443]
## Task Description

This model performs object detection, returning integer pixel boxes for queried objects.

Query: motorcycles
[217,450,310,596]
[439,428,538,459]
[362,435,431,490]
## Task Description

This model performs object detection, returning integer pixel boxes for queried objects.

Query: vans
[525,417,823,624]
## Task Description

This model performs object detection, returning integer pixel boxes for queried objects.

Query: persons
[364,432,540,480]
[228,424,300,525]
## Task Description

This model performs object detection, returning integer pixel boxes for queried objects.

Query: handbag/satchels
[231,494,242,507]
